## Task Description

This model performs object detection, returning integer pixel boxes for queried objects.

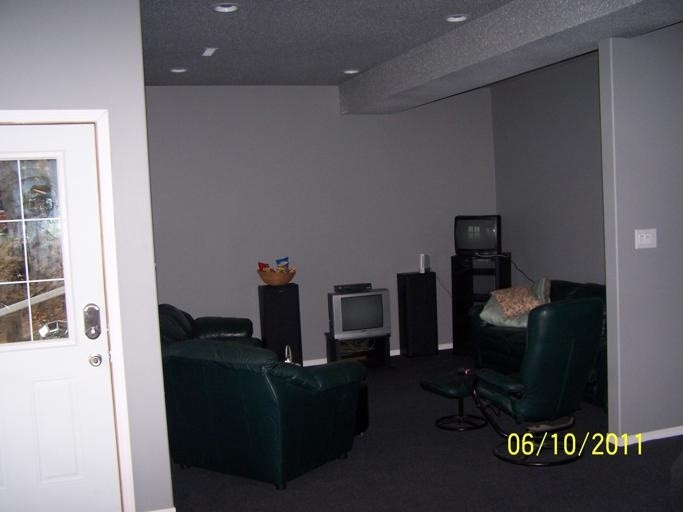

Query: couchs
[158,302,371,490]
[475,280,608,412]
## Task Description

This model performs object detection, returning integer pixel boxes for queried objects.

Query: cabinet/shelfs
[326,331,391,368]
[451,250,511,352]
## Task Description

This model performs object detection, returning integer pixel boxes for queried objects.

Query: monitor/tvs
[328,289,391,339]
[454,215,502,255]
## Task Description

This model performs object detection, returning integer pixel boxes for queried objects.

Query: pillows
[480,277,551,329]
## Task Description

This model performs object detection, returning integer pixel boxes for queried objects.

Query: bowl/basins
[257,270,296,286]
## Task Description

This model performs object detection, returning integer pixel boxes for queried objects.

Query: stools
[422,373,488,433]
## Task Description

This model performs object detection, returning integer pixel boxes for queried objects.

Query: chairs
[461,297,604,467]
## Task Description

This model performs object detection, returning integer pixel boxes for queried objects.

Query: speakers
[258,282,303,366]
[396,272,438,356]
